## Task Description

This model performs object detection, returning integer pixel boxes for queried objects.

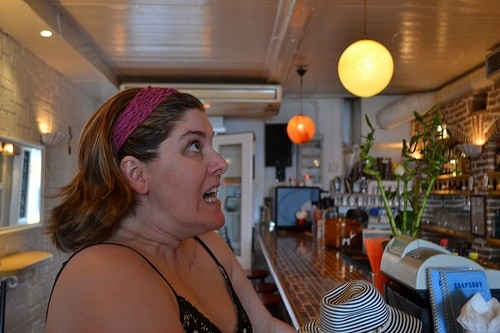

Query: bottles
[324,217,360,252]
[492,208,500,239]
[416,148,500,193]
[332,156,400,195]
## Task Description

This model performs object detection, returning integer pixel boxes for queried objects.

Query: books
[425,266,492,333]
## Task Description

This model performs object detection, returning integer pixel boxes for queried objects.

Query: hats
[298,280,423,333]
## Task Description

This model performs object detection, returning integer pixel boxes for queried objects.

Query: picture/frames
[470,194,487,238]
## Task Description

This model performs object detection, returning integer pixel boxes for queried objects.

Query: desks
[0,250,53,333]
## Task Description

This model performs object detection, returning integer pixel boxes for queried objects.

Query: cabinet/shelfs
[332,173,500,271]
[296,134,324,187]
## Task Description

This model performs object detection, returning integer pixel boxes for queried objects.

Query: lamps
[287,69,314,144]
[338,0,394,98]
[42,132,67,147]
[457,143,482,160]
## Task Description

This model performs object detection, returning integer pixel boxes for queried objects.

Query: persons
[46,87,297,333]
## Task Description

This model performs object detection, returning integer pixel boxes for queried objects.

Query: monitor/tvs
[275,186,320,229]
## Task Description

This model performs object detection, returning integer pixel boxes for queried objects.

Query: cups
[296,218,306,230]
[429,204,472,231]
[370,271,388,301]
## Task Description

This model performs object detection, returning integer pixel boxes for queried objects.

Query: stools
[244,268,281,319]
